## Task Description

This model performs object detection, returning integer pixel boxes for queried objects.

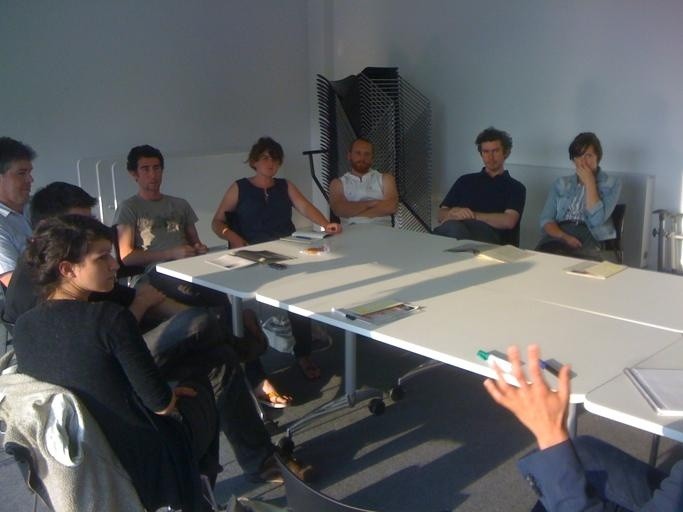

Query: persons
[111,144,294,408]
[431,128,527,247]
[328,139,399,227]
[10,216,217,512]
[484,344,683,512]
[0,136,33,321]
[12,183,312,486]
[211,138,342,381]
[536,132,621,260]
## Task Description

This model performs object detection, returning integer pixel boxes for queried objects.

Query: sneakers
[247,449,317,484]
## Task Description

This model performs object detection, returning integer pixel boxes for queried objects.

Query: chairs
[273,449,374,512]
[110,224,143,279]
[0,365,146,512]
[602,204,627,263]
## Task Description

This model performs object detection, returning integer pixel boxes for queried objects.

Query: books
[334,298,421,323]
[204,249,294,271]
[441,243,535,265]
[623,367,682,417]
[564,260,626,279]
[278,230,332,244]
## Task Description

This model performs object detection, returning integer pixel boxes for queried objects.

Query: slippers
[297,353,320,381]
[252,388,292,408]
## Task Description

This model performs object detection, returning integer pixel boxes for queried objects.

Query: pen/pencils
[293,235,311,240]
[331,308,356,321]
[538,359,559,377]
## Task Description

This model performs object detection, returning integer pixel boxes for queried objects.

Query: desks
[254,259,683,456]
[156,214,457,428]
[584,335,683,468]
[384,239,683,400]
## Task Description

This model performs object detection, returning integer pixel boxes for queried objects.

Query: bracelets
[220,224,229,237]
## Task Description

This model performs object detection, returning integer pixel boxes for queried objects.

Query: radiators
[648,208,683,274]
